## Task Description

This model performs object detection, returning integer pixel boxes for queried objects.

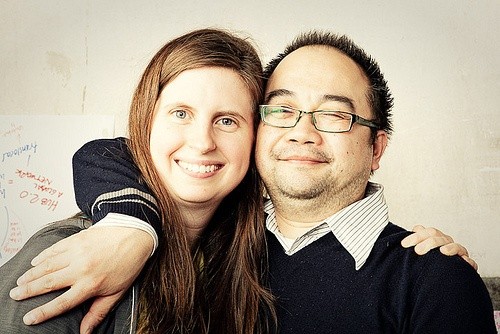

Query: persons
[0,27,478,334]
[8,28,499,334]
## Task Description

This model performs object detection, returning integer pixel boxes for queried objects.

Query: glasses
[259,105,383,133]
